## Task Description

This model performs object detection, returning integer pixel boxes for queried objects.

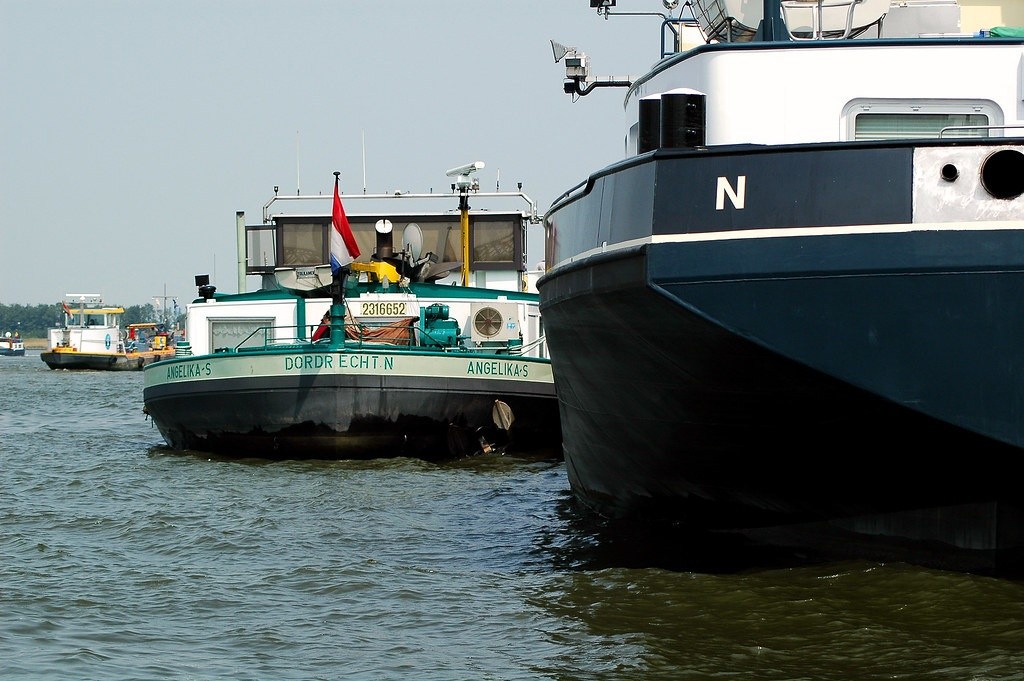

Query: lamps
[195,273,210,286]
[550,38,578,63]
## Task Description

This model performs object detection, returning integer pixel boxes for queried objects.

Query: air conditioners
[470,302,520,343]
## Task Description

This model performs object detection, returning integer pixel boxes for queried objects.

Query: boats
[39,292,176,371]
[536,0,1024,583]
[143,131,564,460]
[0,332,25,356]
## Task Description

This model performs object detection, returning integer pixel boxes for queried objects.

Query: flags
[329,185,362,275]
[62,302,74,320]
